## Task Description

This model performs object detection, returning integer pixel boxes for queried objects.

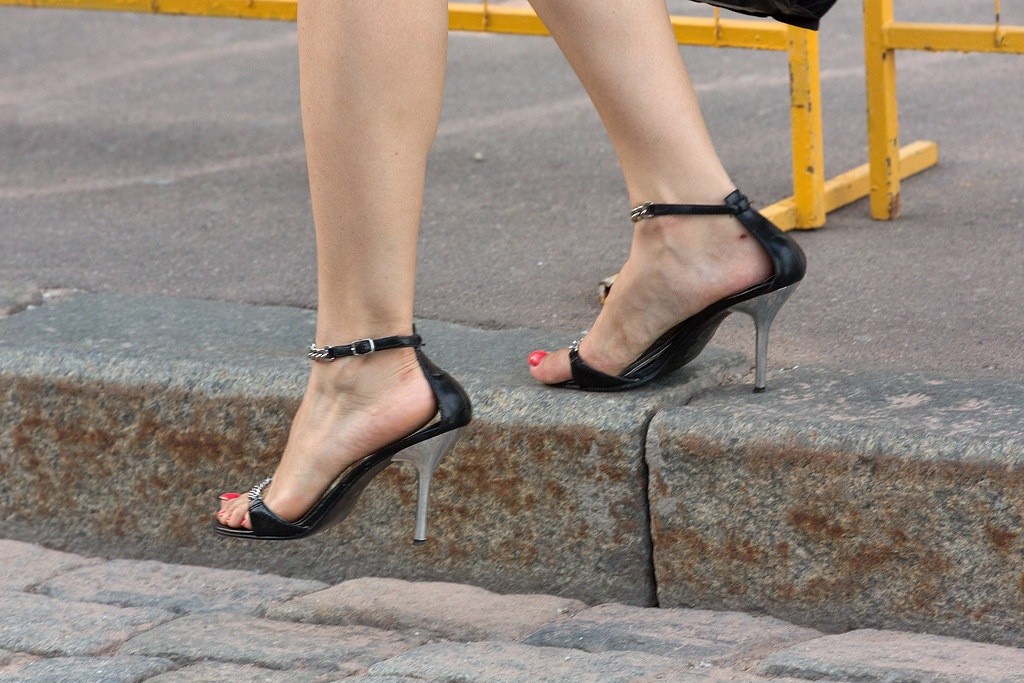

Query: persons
[212,0,808,546]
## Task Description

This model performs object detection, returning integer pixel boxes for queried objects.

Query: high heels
[530,190,807,393]
[213,321,473,545]
